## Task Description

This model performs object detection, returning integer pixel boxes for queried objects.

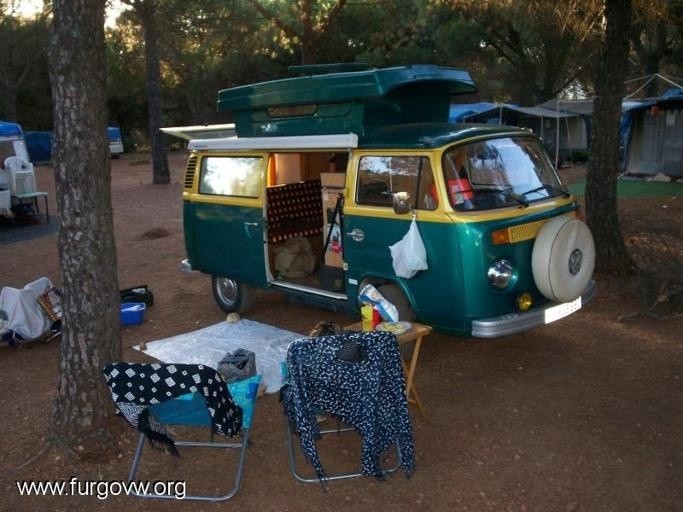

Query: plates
[374,321,411,335]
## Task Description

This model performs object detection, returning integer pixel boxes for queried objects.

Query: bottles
[280,357,287,378]
[360,300,373,330]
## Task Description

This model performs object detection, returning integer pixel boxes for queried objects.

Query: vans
[152,54,604,352]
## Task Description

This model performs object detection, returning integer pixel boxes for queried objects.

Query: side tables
[343,321,433,417]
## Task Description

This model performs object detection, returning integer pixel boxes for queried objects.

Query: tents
[447,88,683,178]
[25,131,56,166]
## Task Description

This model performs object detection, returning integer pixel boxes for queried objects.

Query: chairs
[4,155,49,223]
[267,179,323,244]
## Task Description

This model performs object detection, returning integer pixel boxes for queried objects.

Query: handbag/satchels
[274,236,316,279]
[319,265,346,292]
